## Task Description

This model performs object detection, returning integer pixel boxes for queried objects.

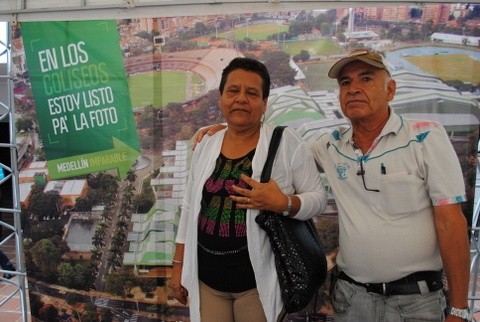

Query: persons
[189,48,475,322]
[172,56,329,322]
[0,250,17,280]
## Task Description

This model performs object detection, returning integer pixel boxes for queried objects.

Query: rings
[248,197,249,203]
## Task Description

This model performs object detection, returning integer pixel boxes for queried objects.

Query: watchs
[447,306,473,322]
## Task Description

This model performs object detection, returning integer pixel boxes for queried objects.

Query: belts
[336,269,444,297]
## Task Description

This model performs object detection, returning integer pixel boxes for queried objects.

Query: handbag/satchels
[254,126,327,314]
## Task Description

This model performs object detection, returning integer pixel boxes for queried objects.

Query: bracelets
[283,196,292,216]
[172,259,182,263]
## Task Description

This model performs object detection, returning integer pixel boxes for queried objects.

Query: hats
[328,48,393,79]
